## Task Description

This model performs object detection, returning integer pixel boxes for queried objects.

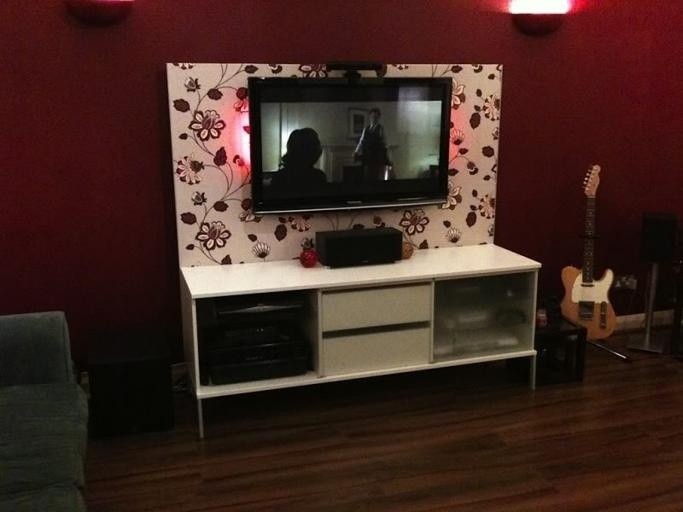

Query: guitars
[558,164,617,340]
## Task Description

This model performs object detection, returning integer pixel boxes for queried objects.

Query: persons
[353,107,393,180]
[271,127,328,190]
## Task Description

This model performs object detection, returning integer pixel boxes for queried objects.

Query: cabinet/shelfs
[177,243,543,439]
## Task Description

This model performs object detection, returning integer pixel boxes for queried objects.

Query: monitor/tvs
[247,75,454,214]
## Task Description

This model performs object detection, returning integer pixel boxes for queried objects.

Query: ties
[370,123,375,131]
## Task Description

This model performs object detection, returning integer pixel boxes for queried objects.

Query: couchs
[2,311,88,512]
